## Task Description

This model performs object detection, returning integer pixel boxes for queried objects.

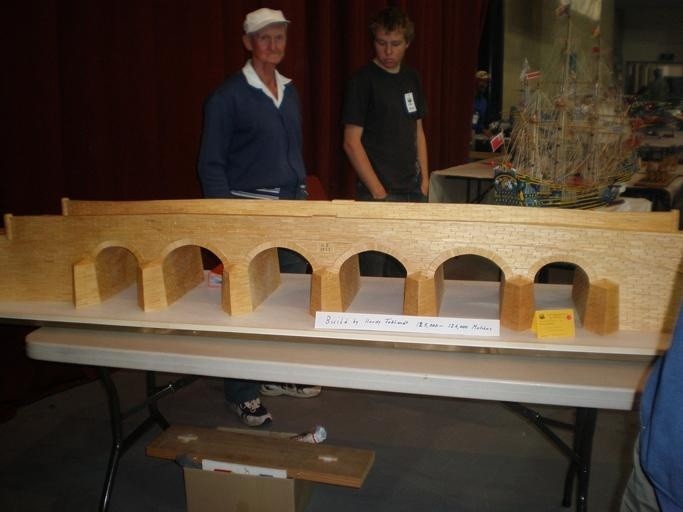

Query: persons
[343,10,429,278]
[617,296,683,512]
[198,8,324,428]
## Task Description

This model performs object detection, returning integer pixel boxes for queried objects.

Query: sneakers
[227,395,272,426]
[259,384,321,398]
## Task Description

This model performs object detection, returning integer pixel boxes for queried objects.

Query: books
[625,57,683,100]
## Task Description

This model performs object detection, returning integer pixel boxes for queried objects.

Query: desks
[427,95,683,213]
[25,326,651,512]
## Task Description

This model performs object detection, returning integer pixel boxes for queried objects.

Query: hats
[243,7,291,35]
